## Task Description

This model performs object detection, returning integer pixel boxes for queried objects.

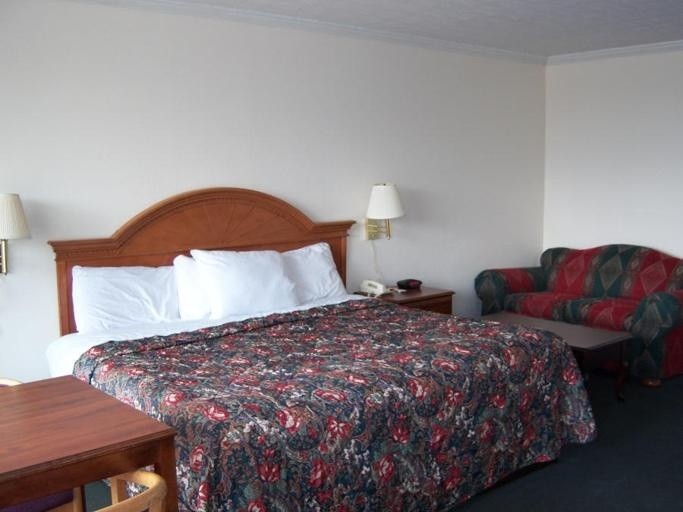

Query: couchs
[475,244,683,387]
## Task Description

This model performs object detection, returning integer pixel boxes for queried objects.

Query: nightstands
[353,286,455,315]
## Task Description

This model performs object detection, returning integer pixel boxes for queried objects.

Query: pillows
[172,253,211,323]
[72,264,195,346]
[190,248,298,327]
[280,242,351,310]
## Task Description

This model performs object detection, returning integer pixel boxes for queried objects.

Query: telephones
[359,279,390,295]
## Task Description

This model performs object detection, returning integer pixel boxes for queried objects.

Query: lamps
[1,192,30,275]
[364,184,405,240]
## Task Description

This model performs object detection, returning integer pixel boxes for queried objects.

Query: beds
[47,187,597,512]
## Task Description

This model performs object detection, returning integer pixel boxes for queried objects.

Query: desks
[0,374,177,512]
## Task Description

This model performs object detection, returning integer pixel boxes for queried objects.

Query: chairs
[0,376,23,387]
[47,468,168,512]
